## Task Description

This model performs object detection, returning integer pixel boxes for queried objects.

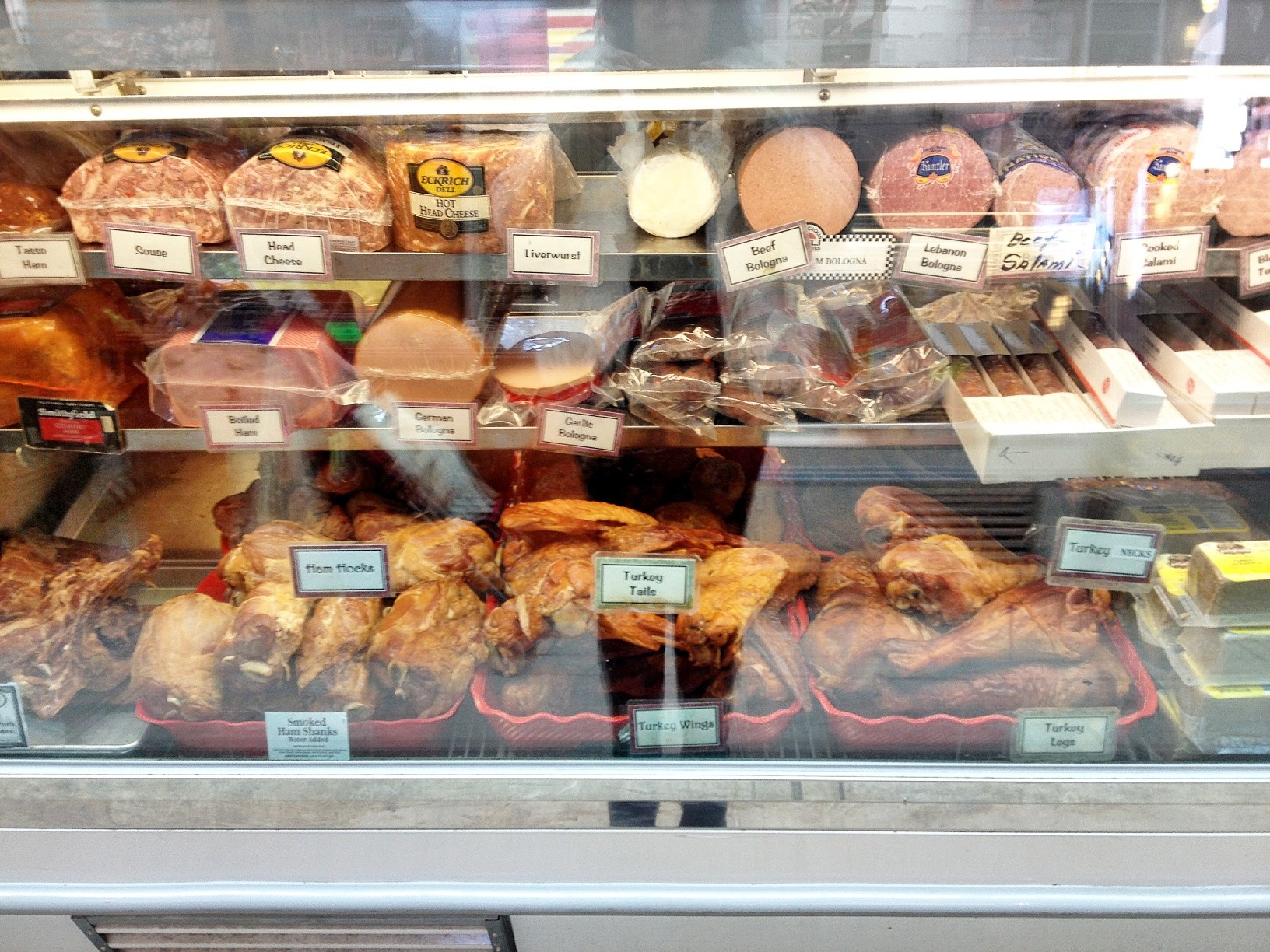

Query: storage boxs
[937,272,1270,486]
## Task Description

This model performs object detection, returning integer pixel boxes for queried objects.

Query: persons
[472,0,932,829]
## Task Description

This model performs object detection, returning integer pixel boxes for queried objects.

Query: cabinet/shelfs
[0,62,1270,889]
